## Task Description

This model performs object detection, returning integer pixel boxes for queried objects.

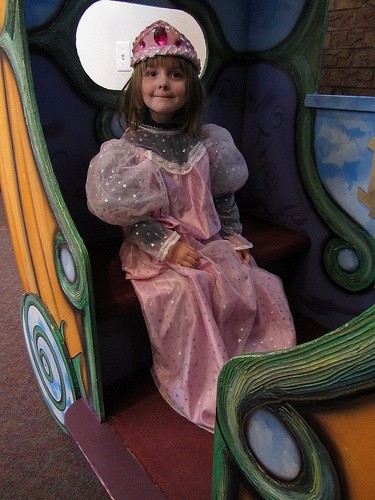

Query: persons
[83,20,300,434]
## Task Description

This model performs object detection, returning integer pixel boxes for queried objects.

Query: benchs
[86,212,298,316]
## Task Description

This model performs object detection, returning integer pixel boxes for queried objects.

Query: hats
[130,18,202,75]
